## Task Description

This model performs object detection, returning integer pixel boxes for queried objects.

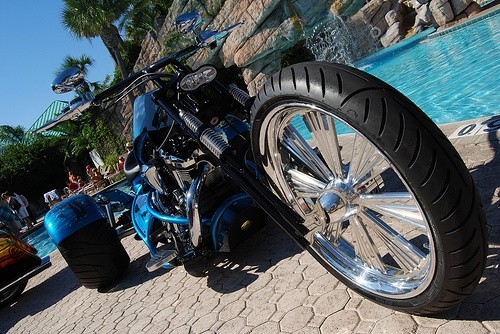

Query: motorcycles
[30,9,488,316]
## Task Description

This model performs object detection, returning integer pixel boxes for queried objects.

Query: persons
[65,142,133,193]
[0,190,38,236]
